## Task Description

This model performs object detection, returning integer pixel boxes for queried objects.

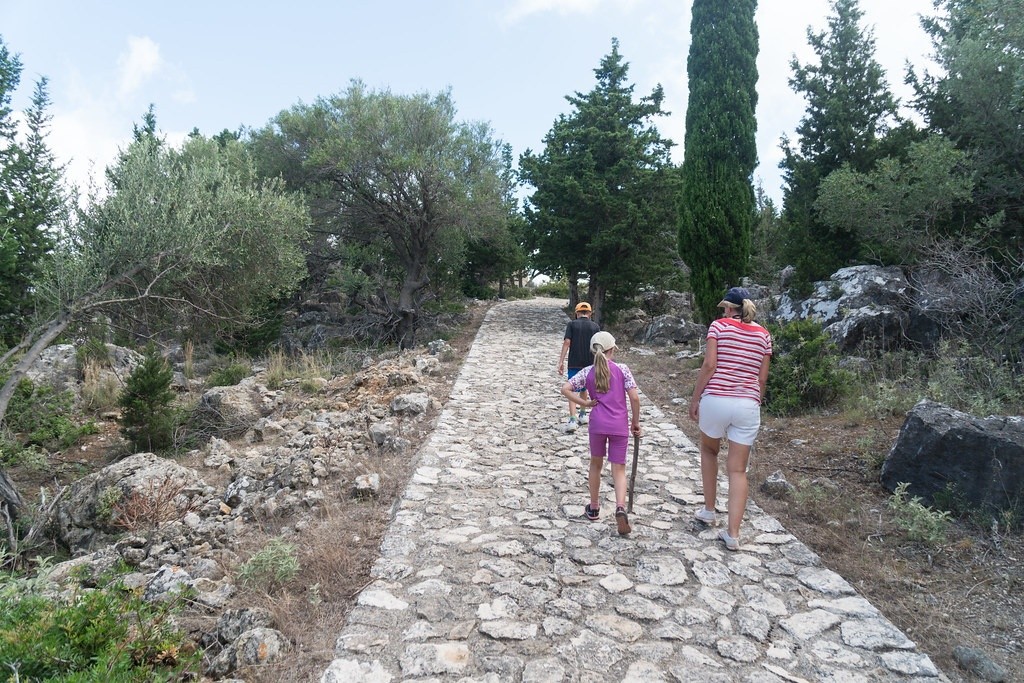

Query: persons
[689,286,773,553]
[561,330,642,535]
[557,302,601,433]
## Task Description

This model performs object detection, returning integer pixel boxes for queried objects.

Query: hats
[590,331,620,352]
[575,302,592,319]
[717,287,750,307]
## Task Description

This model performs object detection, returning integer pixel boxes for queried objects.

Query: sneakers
[615,506,631,534]
[585,504,600,519]
[694,505,716,523]
[577,414,589,423]
[718,529,739,550]
[565,421,577,432]
